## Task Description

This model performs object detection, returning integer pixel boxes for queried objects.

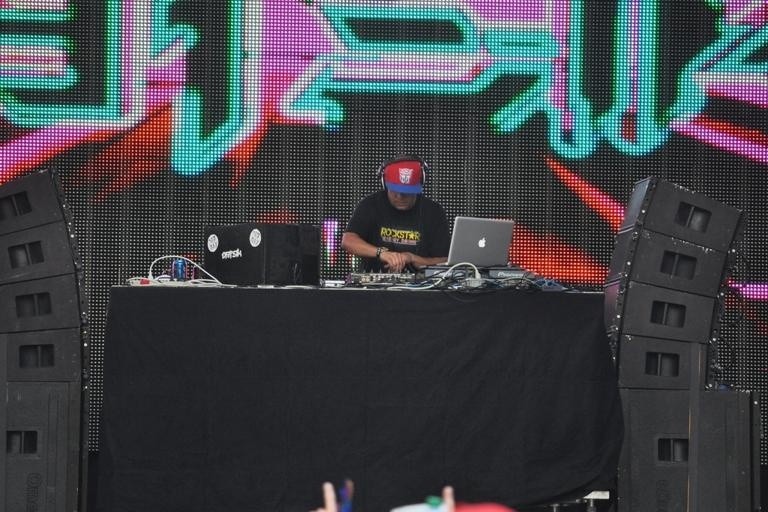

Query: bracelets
[375,246,390,261]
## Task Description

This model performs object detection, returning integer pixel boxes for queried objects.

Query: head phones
[376,155,429,191]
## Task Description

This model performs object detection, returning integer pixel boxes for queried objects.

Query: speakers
[602,176,761,512]
[0,168,91,512]
[204,222,321,287]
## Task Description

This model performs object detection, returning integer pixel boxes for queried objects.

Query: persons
[315,479,455,512]
[339,156,450,279]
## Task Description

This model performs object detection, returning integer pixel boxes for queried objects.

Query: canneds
[172,260,186,280]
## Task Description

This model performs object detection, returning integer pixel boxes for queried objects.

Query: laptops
[419,216,514,268]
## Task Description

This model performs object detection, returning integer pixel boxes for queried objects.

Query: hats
[384,159,423,195]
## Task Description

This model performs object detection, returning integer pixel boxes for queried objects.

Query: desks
[102,281,626,511]
